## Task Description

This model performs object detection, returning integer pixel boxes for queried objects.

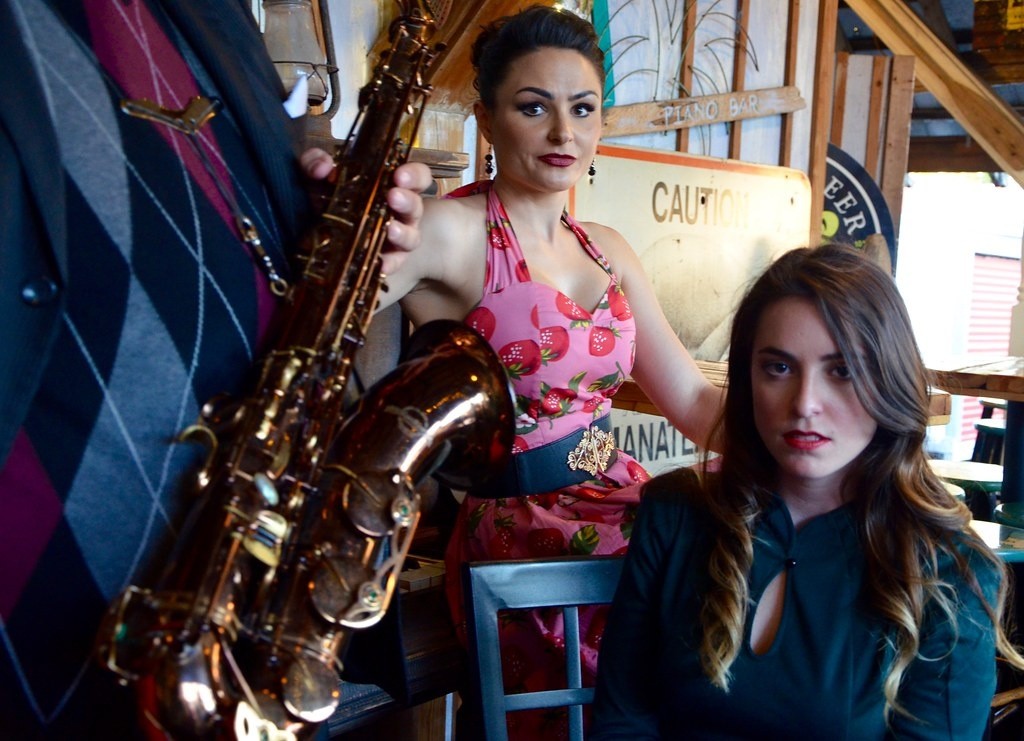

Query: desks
[919,355,1024,501]
[614,359,953,427]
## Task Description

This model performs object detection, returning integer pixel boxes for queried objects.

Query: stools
[977,398,1008,464]
[973,417,1007,462]
[969,519,1024,563]
[926,460,1004,519]
[991,503,1024,645]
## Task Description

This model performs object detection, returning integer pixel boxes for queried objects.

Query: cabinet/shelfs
[325,550,468,729]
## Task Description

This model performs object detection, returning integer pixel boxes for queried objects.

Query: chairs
[474,559,621,741]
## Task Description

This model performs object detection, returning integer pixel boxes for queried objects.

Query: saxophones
[101,1,520,741]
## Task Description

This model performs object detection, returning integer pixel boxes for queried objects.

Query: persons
[373,3,731,741]
[0,0,432,741]
[584,243,1023,741]
